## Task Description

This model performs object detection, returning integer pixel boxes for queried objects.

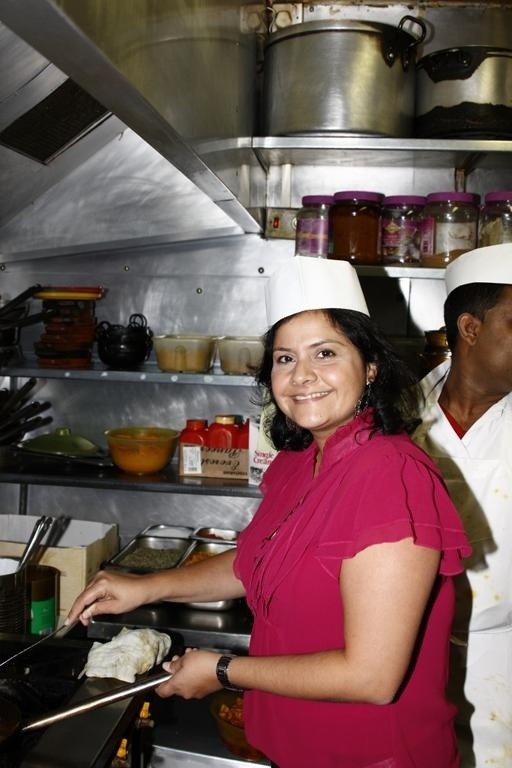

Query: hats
[445,241,512,297]
[262,255,371,327]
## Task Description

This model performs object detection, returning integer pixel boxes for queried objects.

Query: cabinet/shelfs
[0,117,512,504]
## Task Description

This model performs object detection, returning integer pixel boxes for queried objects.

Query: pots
[116,28,256,135]
[260,13,512,140]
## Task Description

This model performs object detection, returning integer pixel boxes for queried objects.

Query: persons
[64,254,475,765]
[393,241,512,765]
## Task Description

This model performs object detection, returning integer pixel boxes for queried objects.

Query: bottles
[179,413,249,450]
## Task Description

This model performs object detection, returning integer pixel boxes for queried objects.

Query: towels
[70,624,175,688]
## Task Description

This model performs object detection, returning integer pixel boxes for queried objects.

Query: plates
[27,282,106,302]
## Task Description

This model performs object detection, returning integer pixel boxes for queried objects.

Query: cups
[0,557,59,638]
[97,327,148,369]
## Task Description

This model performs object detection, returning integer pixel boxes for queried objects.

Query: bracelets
[214,654,245,699]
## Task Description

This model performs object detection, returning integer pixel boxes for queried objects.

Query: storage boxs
[1,511,123,641]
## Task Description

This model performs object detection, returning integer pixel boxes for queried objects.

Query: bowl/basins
[209,690,269,761]
[105,427,179,475]
